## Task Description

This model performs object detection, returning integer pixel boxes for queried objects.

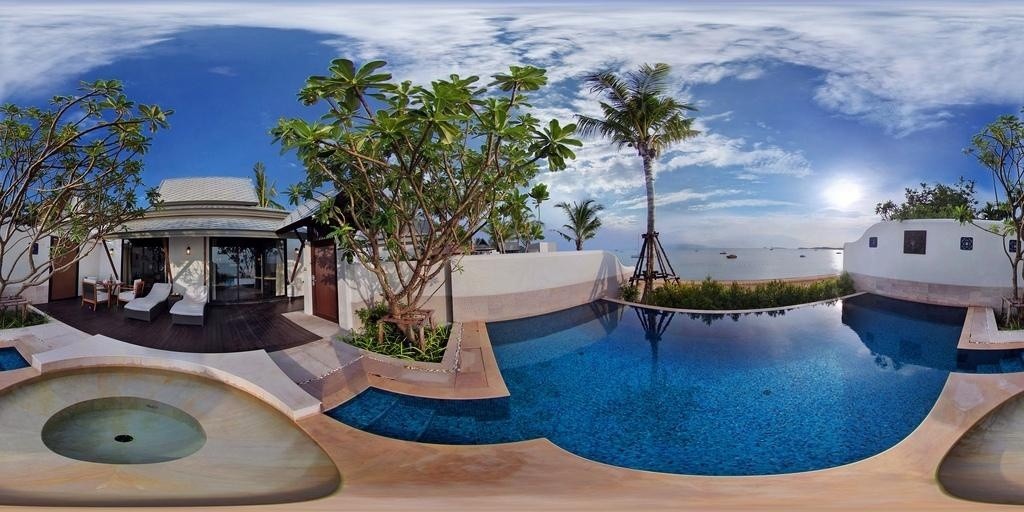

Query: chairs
[74,271,212,328]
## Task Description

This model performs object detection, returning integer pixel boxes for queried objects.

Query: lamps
[108,247,113,255]
[184,246,191,255]
[294,247,299,254]
